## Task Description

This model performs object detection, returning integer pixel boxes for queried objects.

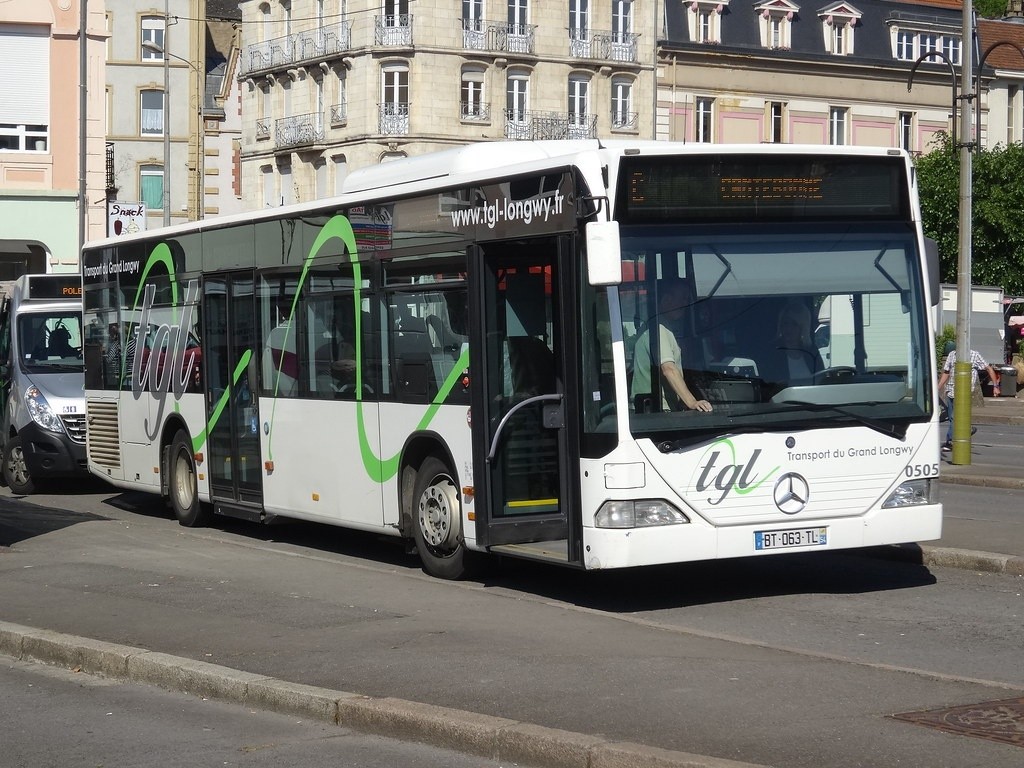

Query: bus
[80,140,943,580]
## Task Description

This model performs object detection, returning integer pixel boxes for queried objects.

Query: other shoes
[943,441,952,451]
[971,427,977,436]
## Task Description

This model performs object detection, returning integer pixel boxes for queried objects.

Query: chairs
[509,336,553,394]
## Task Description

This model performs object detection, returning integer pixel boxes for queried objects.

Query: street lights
[141,39,204,220]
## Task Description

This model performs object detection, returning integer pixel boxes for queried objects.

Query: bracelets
[993,384,998,387]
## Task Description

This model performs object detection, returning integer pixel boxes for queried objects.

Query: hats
[773,297,811,344]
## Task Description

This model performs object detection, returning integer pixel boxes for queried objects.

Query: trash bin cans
[976,364,1019,397]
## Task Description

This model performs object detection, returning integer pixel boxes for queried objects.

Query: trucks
[0,274,82,494]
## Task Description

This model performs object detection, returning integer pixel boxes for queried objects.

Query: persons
[630,294,712,412]
[938,350,1000,452]
[766,302,824,379]
[107,323,136,389]
